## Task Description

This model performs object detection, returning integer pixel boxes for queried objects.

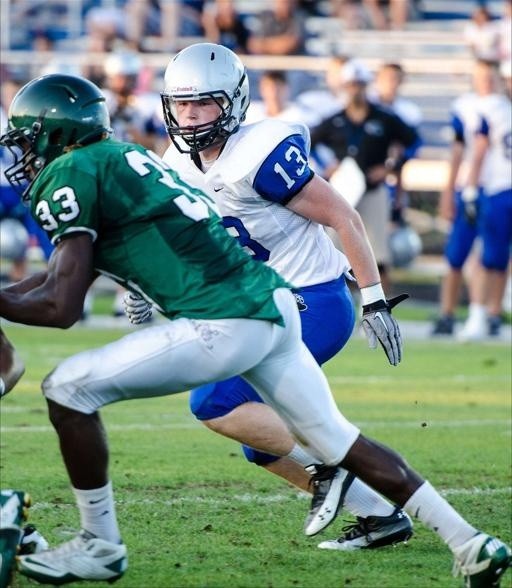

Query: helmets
[160,43,249,154]
[0,74,112,204]
[388,228,422,265]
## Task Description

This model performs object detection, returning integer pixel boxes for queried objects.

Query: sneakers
[430,308,502,343]
[0,489,31,588]
[452,533,512,587]
[303,462,356,536]
[318,506,413,551]
[16,530,127,586]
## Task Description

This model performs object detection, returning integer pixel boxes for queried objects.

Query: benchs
[2,0,512,190]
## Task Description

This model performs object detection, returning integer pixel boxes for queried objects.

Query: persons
[0,70,511,588]
[0,327,49,588]
[120,43,414,550]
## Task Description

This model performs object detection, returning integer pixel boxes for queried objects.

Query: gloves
[362,293,411,365]
[121,292,153,325]
